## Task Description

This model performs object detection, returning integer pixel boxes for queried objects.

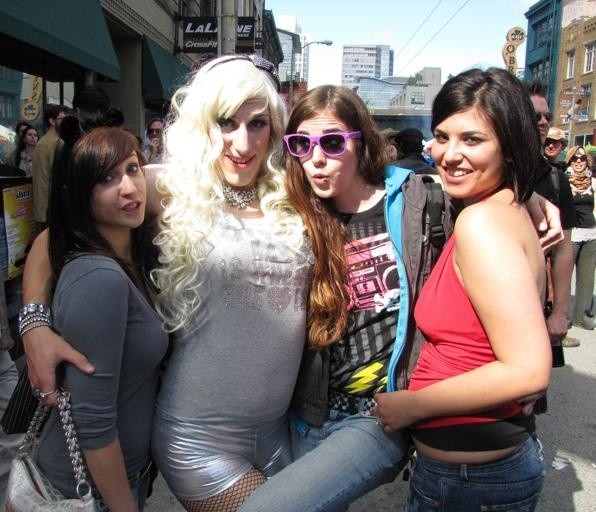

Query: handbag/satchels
[4,455,95,512]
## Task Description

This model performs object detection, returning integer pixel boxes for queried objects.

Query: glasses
[284,131,365,156]
[536,111,552,122]
[148,129,161,133]
[571,155,587,162]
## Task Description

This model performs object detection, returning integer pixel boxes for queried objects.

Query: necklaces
[221,184,259,209]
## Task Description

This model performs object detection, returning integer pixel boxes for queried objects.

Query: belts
[97,460,153,511]
[326,389,379,414]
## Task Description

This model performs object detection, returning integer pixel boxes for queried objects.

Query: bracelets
[17,302,52,337]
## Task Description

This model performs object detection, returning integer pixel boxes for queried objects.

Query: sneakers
[572,318,595,329]
[562,337,580,348]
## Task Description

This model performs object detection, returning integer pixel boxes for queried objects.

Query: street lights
[288,38,333,103]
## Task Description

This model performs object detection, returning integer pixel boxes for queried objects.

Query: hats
[380,128,399,139]
[547,127,568,148]
[394,129,423,142]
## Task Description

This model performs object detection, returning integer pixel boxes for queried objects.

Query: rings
[40,391,58,400]
[376,416,385,426]
[32,389,40,399]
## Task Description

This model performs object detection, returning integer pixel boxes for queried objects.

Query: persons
[35,125,172,512]
[377,129,434,172]
[544,129,596,346]
[14,84,167,239]
[234,86,541,512]
[370,67,554,512]
[18,52,565,512]
